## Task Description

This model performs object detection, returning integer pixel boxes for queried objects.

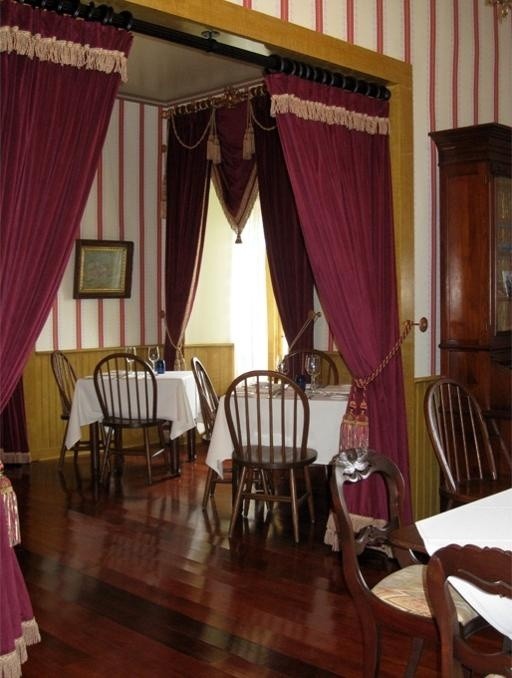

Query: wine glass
[304,355,322,394]
[147,345,160,376]
[125,345,137,375]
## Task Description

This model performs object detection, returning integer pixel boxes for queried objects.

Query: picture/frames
[71,237,134,299]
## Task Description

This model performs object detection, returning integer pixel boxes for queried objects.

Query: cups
[274,353,289,375]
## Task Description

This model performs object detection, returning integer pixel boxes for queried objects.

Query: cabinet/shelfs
[429,118,512,515]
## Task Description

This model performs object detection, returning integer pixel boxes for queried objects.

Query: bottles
[157,359,165,373]
[295,374,305,393]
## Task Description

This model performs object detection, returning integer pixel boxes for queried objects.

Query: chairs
[190,357,273,514]
[50,350,112,470]
[224,369,319,544]
[275,349,339,387]
[328,447,487,677]
[422,378,511,508]
[94,353,170,487]
[424,541,512,678]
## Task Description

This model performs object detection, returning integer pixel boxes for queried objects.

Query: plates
[319,384,352,395]
[326,393,347,401]
[229,391,255,397]
